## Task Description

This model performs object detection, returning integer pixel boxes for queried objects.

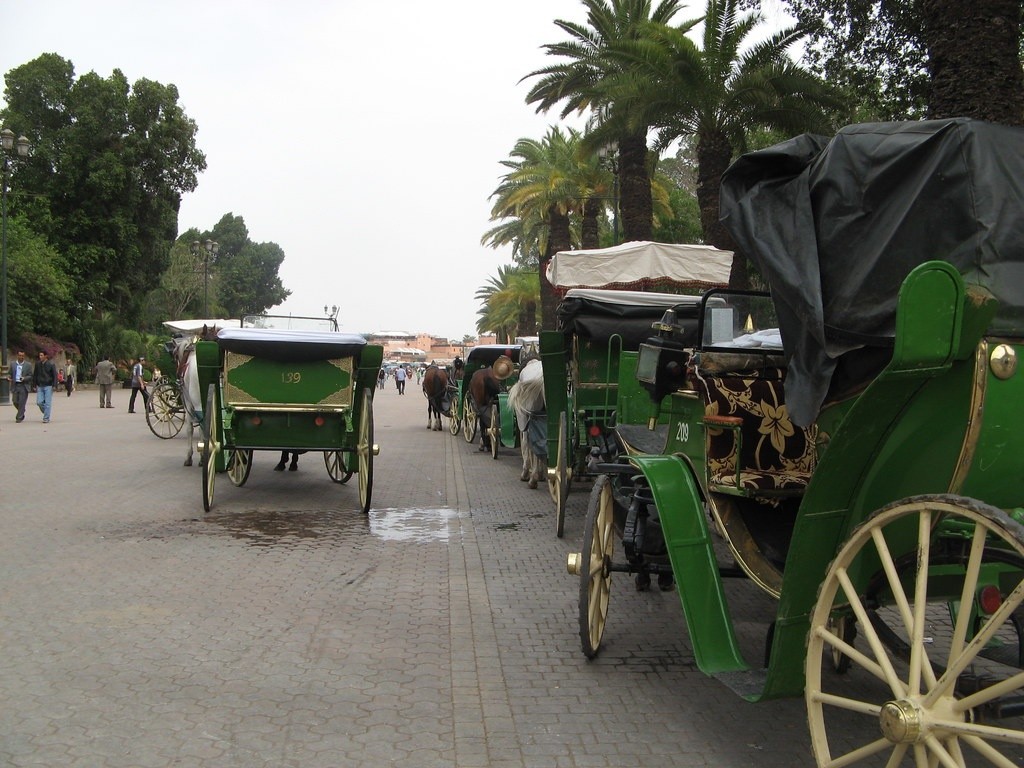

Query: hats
[455,356,460,358]
[493,357,513,380]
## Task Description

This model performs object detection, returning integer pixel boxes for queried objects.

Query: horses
[509,341,546,489]
[450,356,464,387]
[423,360,448,431]
[173,324,299,472]
[469,365,501,451]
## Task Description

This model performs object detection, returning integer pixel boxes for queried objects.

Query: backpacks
[379,370,385,379]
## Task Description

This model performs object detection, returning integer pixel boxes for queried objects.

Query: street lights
[325,305,337,330]
[0,128,32,406]
[192,238,219,317]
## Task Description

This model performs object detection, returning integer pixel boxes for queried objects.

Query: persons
[31,350,56,423]
[393,368,400,389]
[151,366,162,384]
[64,359,74,397]
[127,356,152,414]
[415,367,422,386]
[94,353,117,408]
[397,364,406,395]
[6,349,32,423]
[379,368,385,390]
[58,369,76,392]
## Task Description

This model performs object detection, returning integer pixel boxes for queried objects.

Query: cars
[380,361,430,374]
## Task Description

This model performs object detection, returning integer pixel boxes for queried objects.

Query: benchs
[684,352,831,503]
[565,317,699,413]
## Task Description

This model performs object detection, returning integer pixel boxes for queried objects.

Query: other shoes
[100,406,104,408]
[128,410,136,413]
[16,415,24,423]
[149,409,153,412]
[43,418,49,423]
[106,406,114,408]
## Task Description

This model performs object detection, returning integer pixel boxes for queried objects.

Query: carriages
[144,311,384,513]
[421,113,1024,768]
